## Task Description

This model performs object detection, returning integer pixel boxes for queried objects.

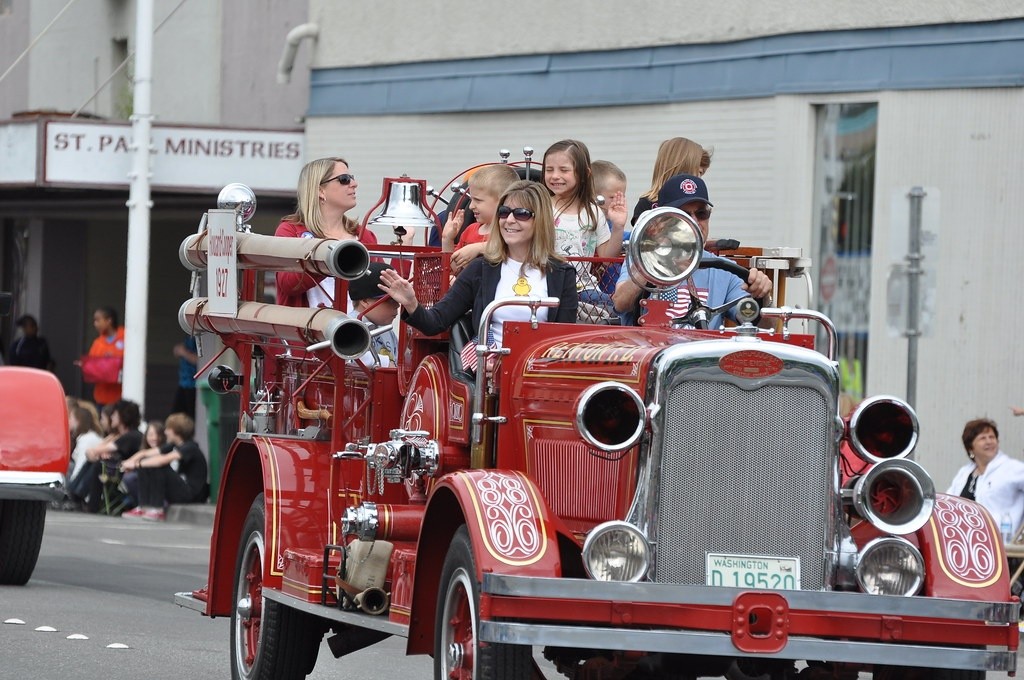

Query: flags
[459,330,497,374]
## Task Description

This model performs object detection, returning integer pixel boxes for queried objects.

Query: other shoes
[121,506,144,520]
[142,508,165,523]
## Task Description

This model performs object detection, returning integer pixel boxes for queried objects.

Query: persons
[262,156,416,402]
[377,179,578,396]
[630,136,715,228]
[45,304,209,517]
[3,313,59,371]
[540,139,629,305]
[344,261,412,368]
[590,159,631,295]
[945,416,1024,545]
[612,174,786,329]
[441,162,522,285]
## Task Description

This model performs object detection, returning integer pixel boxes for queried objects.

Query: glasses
[496,205,535,221]
[320,174,355,185]
[682,208,712,221]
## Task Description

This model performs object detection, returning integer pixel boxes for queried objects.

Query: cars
[0,366,71,588]
[177,147,1024,680]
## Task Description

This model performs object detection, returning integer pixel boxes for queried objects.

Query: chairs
[449,313,476,432]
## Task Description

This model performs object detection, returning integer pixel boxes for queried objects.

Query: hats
[348,262,399,300]
[657,174,714,209]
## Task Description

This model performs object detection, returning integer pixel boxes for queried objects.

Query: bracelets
[761,296,773,308]
